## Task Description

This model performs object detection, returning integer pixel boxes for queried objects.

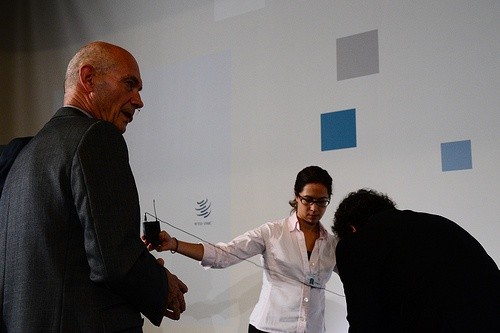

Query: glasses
[298,194,330,207]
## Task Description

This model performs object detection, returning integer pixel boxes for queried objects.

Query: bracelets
[170,236,178,254]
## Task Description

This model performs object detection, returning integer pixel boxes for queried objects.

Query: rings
[172,302,180,309]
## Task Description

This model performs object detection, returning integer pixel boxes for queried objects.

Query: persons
[142,165,342,333]
[1,41,189,333]
[333,188,500,333]
